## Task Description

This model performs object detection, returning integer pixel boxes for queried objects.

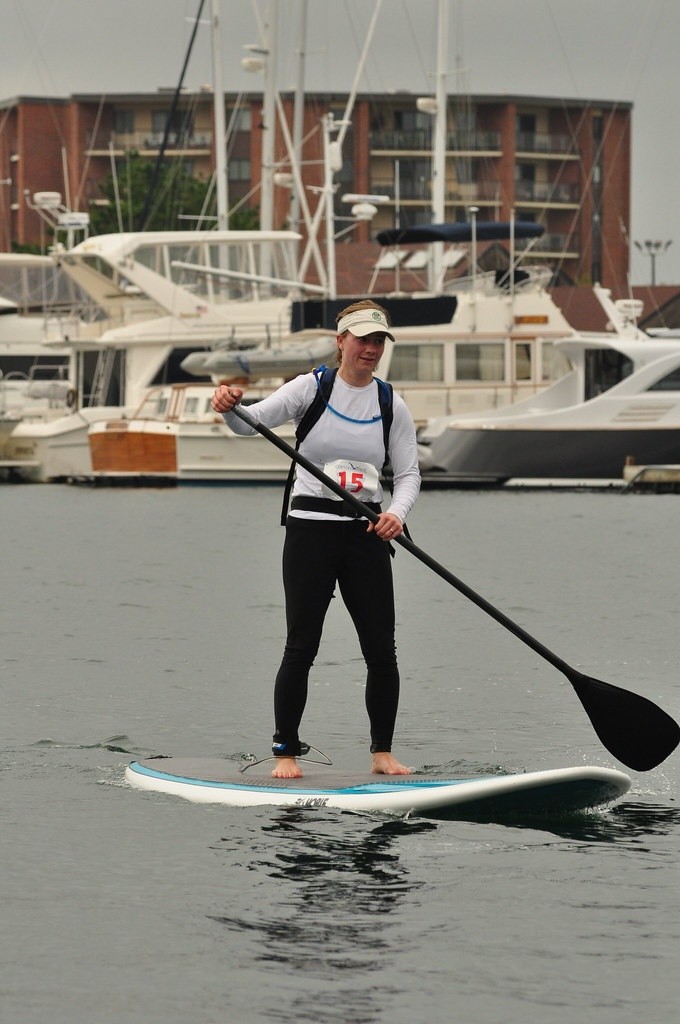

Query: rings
[389,529,395,534]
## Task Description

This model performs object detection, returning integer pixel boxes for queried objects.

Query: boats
[3,8,677,486]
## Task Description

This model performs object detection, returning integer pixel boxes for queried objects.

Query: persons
[212,298,423,780]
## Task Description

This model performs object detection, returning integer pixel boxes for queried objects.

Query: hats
[335,309,395,342]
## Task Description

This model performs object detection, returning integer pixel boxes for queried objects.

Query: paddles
[228,393,680,773]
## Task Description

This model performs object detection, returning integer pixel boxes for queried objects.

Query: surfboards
[121,757,630,824]
